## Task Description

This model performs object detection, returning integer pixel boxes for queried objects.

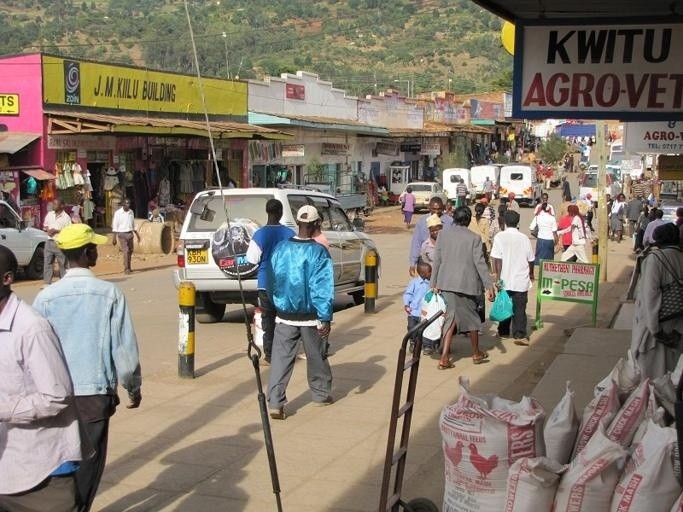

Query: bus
[609,140,646,181]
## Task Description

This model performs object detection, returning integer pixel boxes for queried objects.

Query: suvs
[176,187,381,323]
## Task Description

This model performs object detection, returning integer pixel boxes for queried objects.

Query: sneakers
[269,408,286,420]
[313,396,332,407]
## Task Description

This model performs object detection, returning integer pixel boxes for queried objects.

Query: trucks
[301,184,375,232]
[442,168,477,204]
[470,165,499,200]
[587,164,619,175]
[500,165,544,208]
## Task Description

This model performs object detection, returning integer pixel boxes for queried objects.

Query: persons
[33,223,142,512]
[563,142,591,172]
[506,148,523,162]
[377,184,389,206]
[39,198,71,289]
[537,161,553,190]
[675,207,682,228]
[248,198,334,419]
[402,188,416,229]
[481,176,494,203]
[148,195,167,223]
[403,197,535,371]
[1,245,83,512]
[488,157,494,164]
[505,192,520,230]
[424,166,439,180]
[631,222,683,378]
[529,193,597,266]
[112,198,140,275]
[606,172,666,254]
[561,175,570,202]
[456,178,469,206]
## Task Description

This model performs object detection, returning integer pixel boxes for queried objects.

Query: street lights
[219,31,230,78]
[393,79,409,98]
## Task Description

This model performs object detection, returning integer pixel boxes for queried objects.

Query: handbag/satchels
[658,278,682,322]
[530,225,538,238]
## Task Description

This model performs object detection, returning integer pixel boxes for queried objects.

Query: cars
[491,164,506,168]
[0,201,55,280]
[399,182,448,211]
[579,170,621,203]
[646,154,659,170]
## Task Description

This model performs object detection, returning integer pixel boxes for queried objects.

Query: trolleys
[377,310,445,511]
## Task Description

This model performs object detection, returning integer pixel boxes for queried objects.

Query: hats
[56,224,107,250]
[296,205,320,222]
[425,213,442,228]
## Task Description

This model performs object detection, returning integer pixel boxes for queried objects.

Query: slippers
[472,353,488,364]
[514,338,528,345]
[438,362,454,370]
